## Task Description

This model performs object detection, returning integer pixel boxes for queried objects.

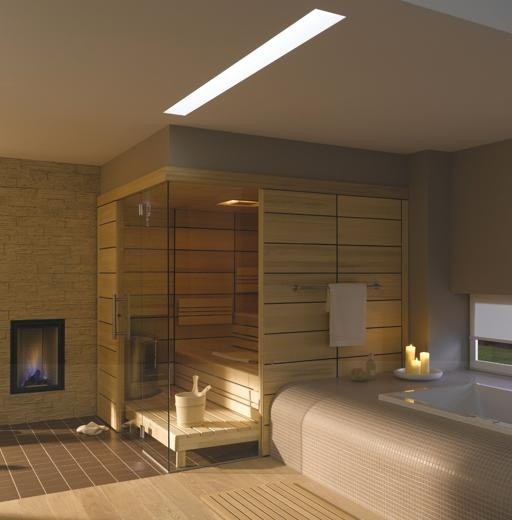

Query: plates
[394,368,444,381]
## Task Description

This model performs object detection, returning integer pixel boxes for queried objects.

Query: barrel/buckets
[174,392,207,428]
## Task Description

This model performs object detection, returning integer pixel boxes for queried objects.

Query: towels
[209,347,259,365]
[325,280,369,349]
[72,420,112,437]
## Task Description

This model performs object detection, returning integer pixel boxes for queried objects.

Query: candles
[410,358,421,375]
[420,351,430,375]
[405,346,417,371]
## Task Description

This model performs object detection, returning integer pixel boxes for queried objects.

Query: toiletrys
[367,353,378,380]
[351,366,368,380]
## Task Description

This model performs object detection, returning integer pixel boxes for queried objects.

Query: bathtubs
[377,380,512,437]
